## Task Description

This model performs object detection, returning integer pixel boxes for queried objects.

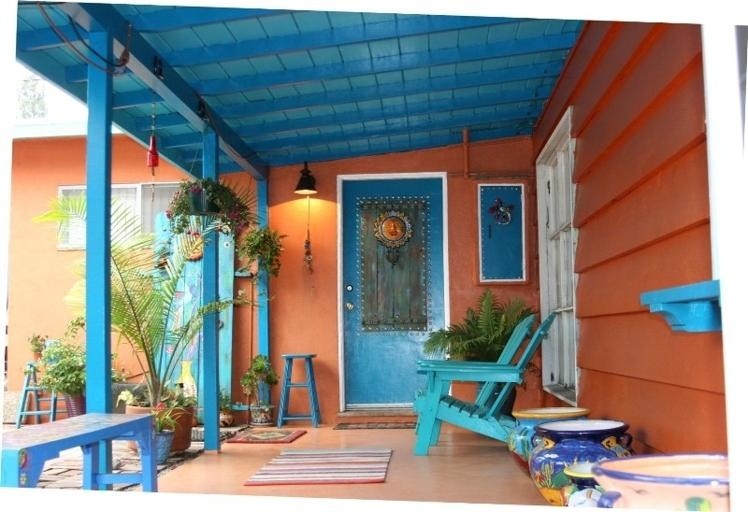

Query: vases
[507,406,729,510]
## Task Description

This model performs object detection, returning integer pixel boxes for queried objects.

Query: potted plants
[217,389,237,427]
[33,194,263,453]
[137,397,187,465]
[27,313,86,417]
[424,288,541,415]
[240,354,277,427]
[162,178,251,238]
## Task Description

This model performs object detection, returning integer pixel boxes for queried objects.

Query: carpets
[193,420,417,485]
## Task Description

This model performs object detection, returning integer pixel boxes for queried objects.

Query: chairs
[412,312,558,453]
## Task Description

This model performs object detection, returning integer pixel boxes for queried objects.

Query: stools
[277,353,322,426]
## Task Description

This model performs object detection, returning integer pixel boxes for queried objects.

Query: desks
[0,412,159,489]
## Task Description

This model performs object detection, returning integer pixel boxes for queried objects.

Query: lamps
[294,160,318,194]
